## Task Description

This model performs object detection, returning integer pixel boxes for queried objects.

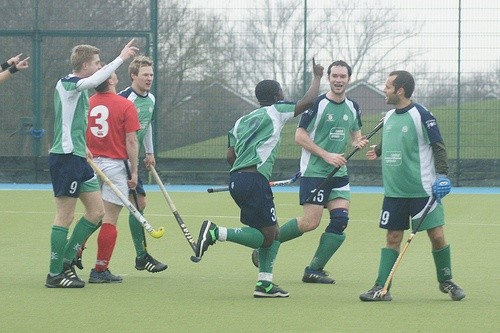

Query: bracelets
[0,61,10,71]
[8,65,20,75]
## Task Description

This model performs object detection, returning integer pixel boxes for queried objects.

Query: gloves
[432,176,451,204]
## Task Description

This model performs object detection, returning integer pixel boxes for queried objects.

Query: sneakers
[358,284,393,302]
[88,268,123,283]
[252,249,258,268]
[196,220,217,258]
[254,281,290,297]
[45,272,85,289]
[135,253,168,272]
[63,262,80,282]
[302,266,336,284]
[76,257,83,270]
[439,280,466,301]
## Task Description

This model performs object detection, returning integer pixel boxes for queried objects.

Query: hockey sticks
[306,117,387,200]
[144,156,196,256]
[83,154,165,238]
[207,172,301,195]
[373,196,438,298]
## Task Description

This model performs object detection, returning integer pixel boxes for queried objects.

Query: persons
[359,71,466,302]
[0,52,30,81]
[67,55,168,284]
[195,56,325,298]
[252,59,369,285]
[44,38,140,288]
